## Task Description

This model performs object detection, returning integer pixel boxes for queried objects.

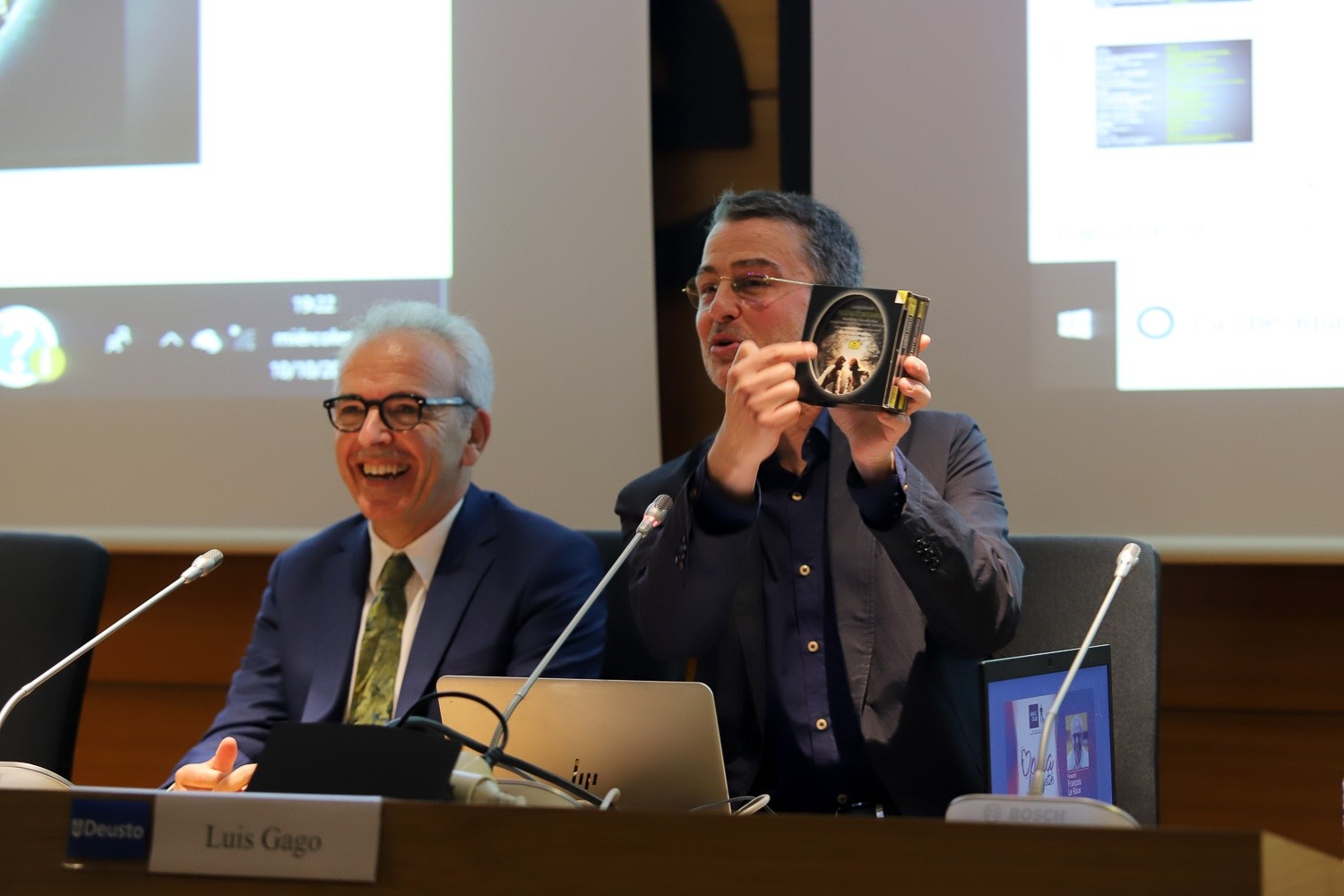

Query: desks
[0,785,1344,896]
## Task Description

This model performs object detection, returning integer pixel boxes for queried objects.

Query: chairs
[992,533,1163,830]
[0,527,110,784]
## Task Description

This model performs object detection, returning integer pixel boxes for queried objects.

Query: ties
[347,554,414,726]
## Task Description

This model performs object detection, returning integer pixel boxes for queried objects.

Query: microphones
[946,543,1144,834]
[0,547,225,788]
[452,492,674,808]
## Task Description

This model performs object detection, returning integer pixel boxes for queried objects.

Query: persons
[818,356,871,394]
[157,303,608,792]
[612,186,1028,816]
[1067,714,1090,770]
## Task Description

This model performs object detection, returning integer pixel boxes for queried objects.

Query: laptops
[977,644,1118,813]
[436,675,731,815]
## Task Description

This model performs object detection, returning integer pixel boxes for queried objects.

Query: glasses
[681,272,817,312]
[324,395,477,432]
